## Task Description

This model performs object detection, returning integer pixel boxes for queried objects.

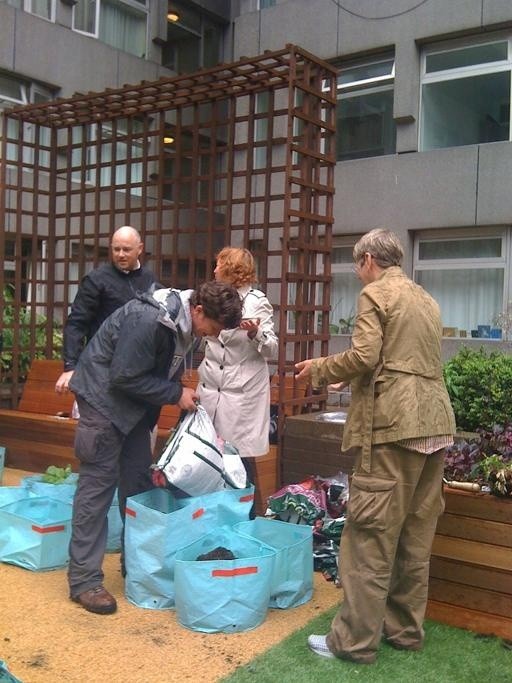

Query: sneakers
[70,585,117,614]
[307,634,334,657]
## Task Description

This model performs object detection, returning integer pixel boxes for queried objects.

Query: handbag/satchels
[124,481,314,634]
[0,472,123,572]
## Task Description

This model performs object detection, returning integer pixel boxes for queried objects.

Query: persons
[194,246,278,520]
[56,223,159,455]
[293,226,456,664]
[68,280,243,615]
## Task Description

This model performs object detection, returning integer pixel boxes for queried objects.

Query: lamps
[164,135,176,144]
[168,11,179,22]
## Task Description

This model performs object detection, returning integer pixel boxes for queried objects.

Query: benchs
[0,361,310,516]
